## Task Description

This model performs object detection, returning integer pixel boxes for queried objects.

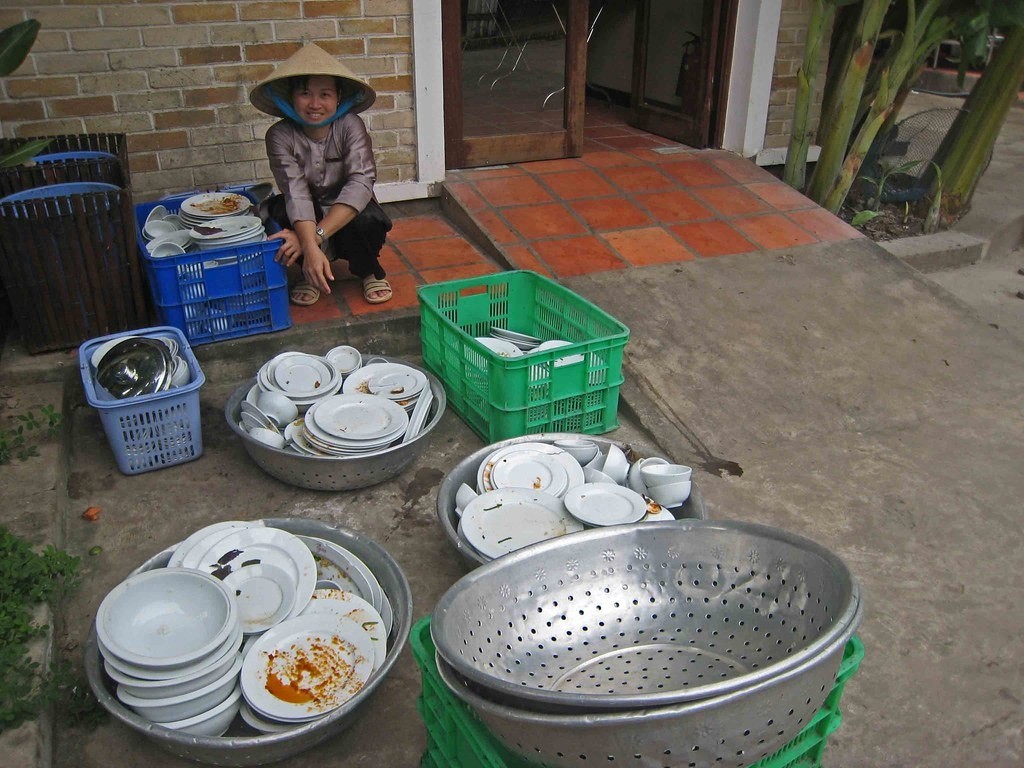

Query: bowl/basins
[248,427,286,449]
[94,371,118,401]
[255,391,299,427]
[553,439,693,509]
[145,220,175,240]
[83,517,413,768]
[454,482,479,519]
[314,580,342,592]
[170,355,190,387]
[95,566,245,737]
[151,241,186,258]
[324,345,390,377]
[436,431,708,565]
[177,259,270,336]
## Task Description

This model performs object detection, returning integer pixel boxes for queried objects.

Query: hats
[249,42,376,118]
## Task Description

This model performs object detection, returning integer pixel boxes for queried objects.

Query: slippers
[362,277,392,304]
[290,271,320,306]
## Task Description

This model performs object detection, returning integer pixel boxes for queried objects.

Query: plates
[119,401,194,468]
[444,326,609,427]
[166,520,394,733]
[457,442,676,562]
[90,335,179,369]
[238,351,434,457]
[141,192,268,264]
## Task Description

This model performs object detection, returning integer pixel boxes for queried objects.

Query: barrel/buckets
[0,151,141,348]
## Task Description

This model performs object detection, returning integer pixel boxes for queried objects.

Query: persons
[247,42,393,304]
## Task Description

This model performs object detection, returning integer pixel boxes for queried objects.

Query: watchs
[316,227,326,242]
